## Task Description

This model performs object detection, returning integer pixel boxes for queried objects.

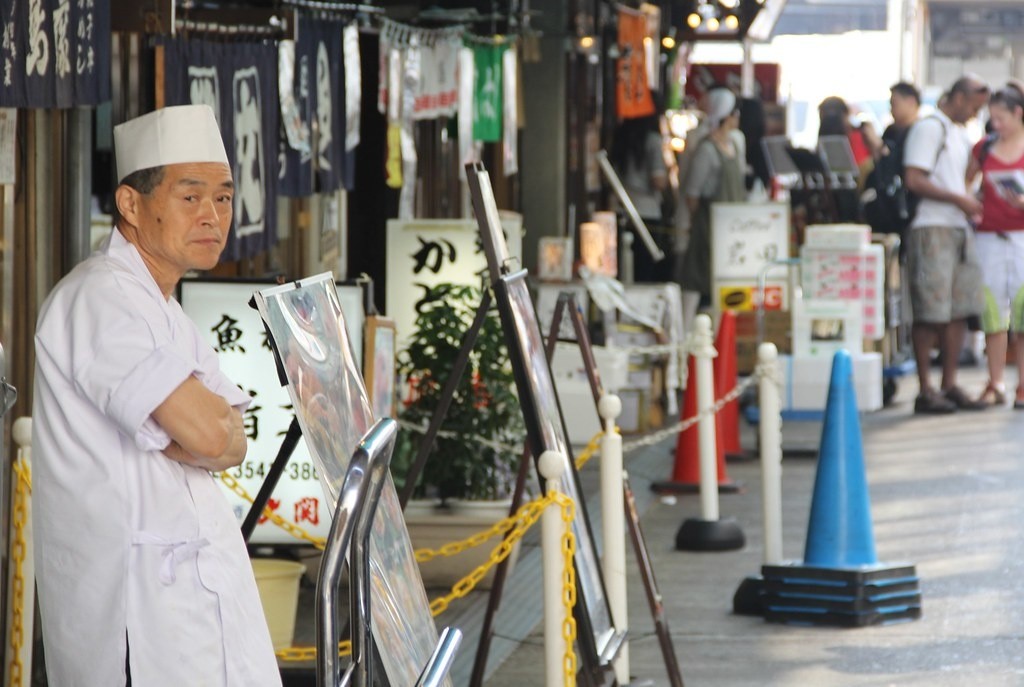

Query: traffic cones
[713,311,754,463]
[650,352,738,497]
[755,352,925,624]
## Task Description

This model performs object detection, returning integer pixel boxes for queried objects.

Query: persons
[673,79,1000,317]
[605,89,673,282]
[29,103,285,687]
[966,80,1023,409]
[898,69,991,417]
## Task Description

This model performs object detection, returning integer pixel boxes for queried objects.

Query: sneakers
[941,388,983,408]
[978,383,1006,405]
[915,388,958,413]
[1013,386,1024,406]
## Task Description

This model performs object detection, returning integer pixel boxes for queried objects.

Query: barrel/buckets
[250,558,307,657]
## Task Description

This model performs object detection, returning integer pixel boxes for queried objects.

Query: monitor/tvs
[818,134,859,177]
[763,134,799,180]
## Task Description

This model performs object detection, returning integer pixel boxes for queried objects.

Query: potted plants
[386,262,536,589]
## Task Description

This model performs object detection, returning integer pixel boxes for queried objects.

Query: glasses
[969,84,990,93]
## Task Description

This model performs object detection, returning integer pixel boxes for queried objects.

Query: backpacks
[864,116,947,229]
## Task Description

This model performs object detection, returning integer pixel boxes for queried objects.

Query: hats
[700,86,736,124]
[818,97,847,111]
[113,104,231,184]
